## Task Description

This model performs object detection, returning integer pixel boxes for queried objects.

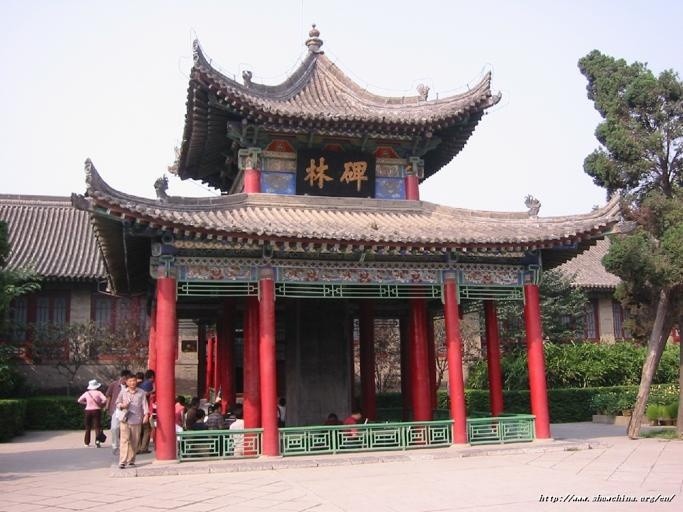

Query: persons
[324,413,342,425]
[341,408,364,439]
[357,409,368,431]
[176,393,245,457]
[276,398,286,428]
[78,368,155,468]
[184,343,195,352]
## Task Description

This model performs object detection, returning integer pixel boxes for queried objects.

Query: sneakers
[119,463,135,469]
[137,450,151,454]
[84,441,101,448]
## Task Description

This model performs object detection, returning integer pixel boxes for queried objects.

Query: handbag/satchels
[97,433,106,442]
[99,403,107,408]
[119,409,129,422]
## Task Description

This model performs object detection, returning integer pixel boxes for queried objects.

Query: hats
[86,379,101,390]
